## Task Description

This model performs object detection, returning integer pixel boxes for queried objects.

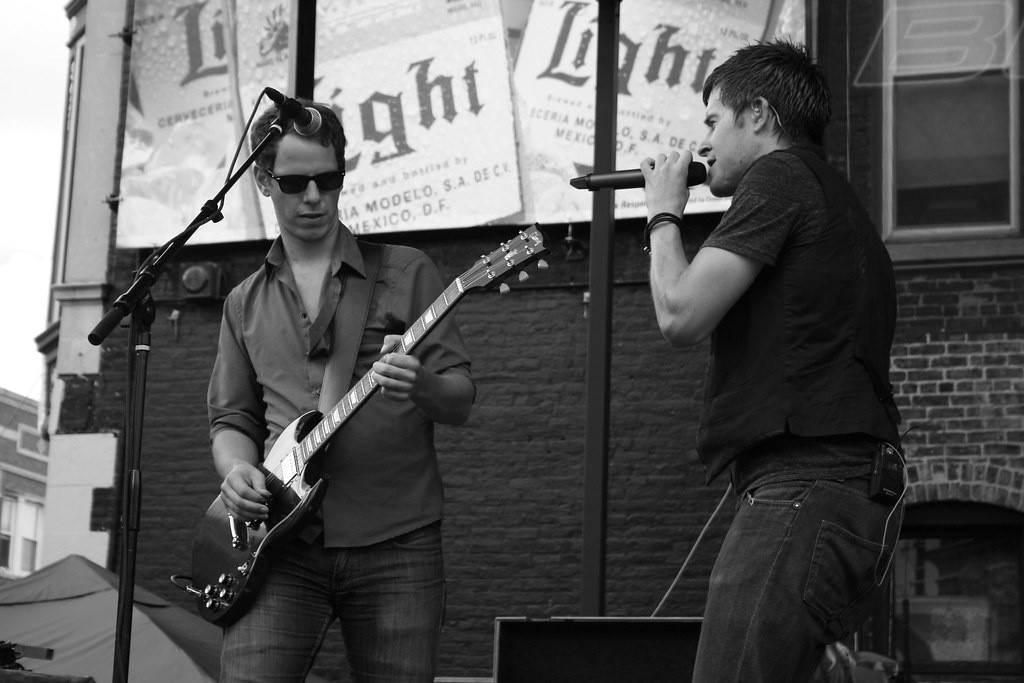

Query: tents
[0,554,325,683]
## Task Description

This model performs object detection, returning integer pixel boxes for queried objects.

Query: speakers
[492,612,704,683]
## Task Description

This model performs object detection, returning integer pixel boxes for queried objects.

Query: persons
[206,97,476,683]
[641,41,903,683]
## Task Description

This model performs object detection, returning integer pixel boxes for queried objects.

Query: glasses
[266,168,344,195]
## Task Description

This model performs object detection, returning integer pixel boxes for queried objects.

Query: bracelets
[643,213,685,256]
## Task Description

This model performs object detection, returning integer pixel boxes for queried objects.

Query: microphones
[265,87,323,138]
[569,160,707,192]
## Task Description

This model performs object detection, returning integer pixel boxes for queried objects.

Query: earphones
[753,106,761,122]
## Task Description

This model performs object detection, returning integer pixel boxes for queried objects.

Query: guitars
[185,222,551,626]
